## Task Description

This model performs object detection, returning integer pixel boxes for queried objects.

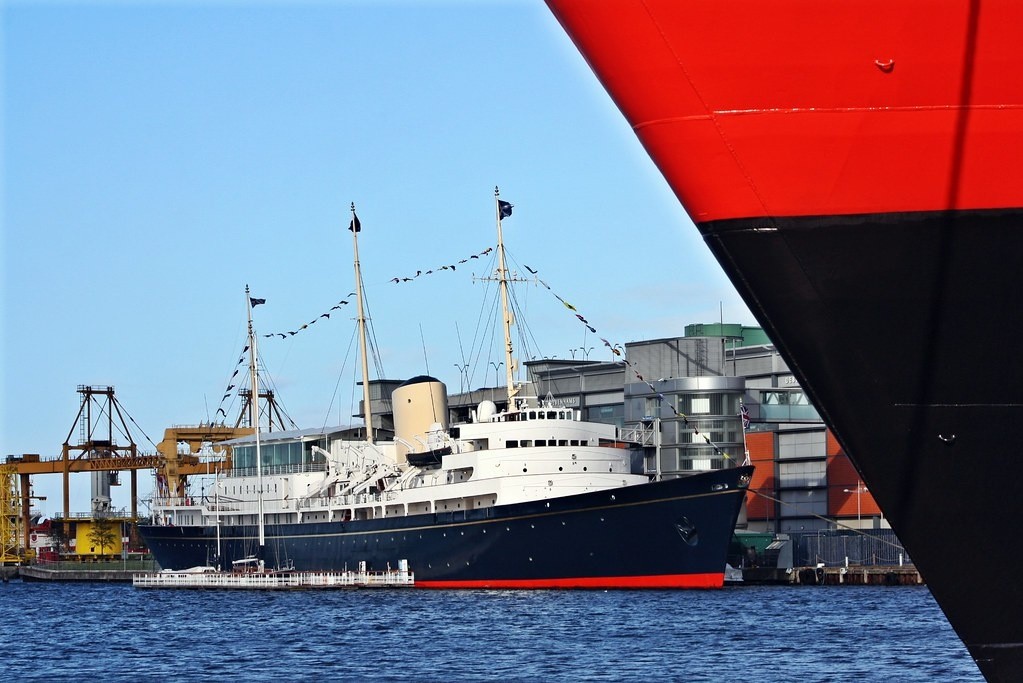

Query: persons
[749,546,760,568]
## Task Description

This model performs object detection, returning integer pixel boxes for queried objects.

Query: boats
[545,0,1023,683]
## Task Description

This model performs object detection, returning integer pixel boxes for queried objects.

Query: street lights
[490,362,504,387]
[454,364,469,393]
[580,347,594,361]
[544,356,557,359]
[569,350,578,360]
[608,344,625,362]
[844,480,860,529]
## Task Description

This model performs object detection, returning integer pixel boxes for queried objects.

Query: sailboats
[131,186,754,588]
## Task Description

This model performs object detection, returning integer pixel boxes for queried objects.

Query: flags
[348,214,361,232]
[741,404,750,429]
[264,293,357,339]
[389,248,493,284]
[498,200,512,221]
[208,346,249,430]
[250,297,266,308]
[524,265,729,460]
